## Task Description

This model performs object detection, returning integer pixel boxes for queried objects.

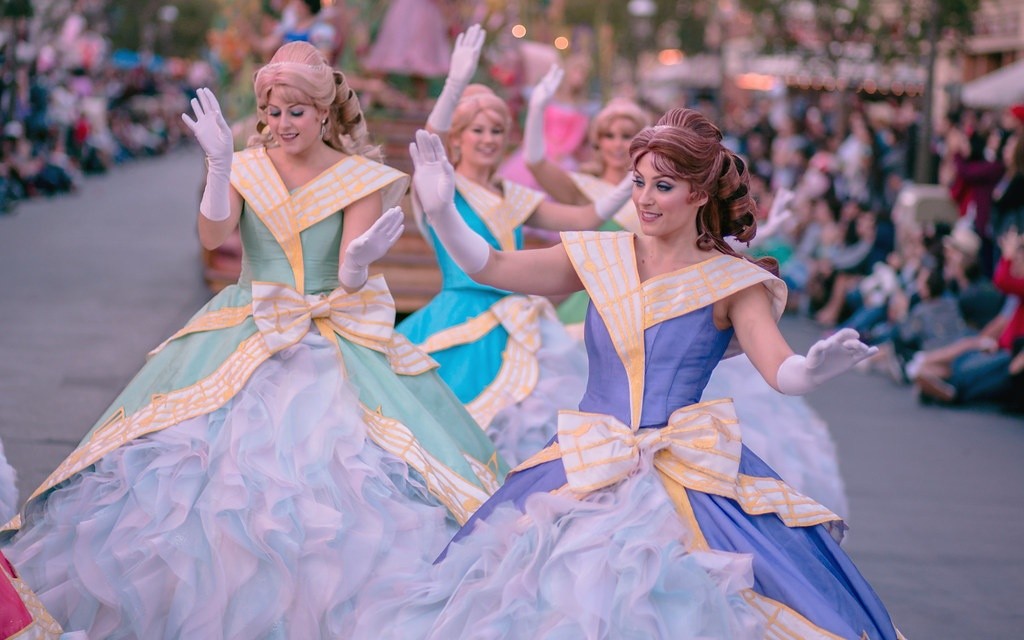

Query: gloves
[522,64,564,163]
[426,22,486,129]
[724,186,795,255]
[406,128,486,274]
[181,88,235,222]
[776,328,877,395]
[339,206,404,285]
[594,172,635,220]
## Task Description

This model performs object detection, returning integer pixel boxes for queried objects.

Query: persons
[0,0,1024,640]
